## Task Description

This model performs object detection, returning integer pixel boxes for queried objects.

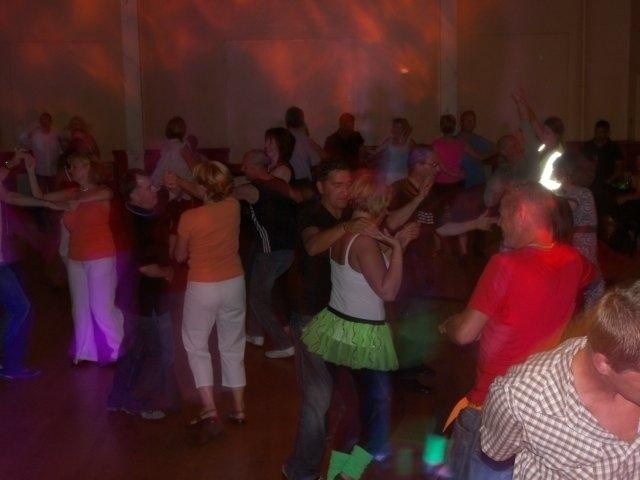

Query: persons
[0,103,639,479]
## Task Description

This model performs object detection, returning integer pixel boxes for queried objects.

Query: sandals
[228,412,246,424]
[189,409,218,426]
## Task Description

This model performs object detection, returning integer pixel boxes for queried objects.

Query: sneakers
[264,340,296,358]
[1,368,40,381]
[106,397,165,420]
[245,324,265,346]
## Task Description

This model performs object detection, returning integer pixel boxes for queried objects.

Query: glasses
[423,161,439,167]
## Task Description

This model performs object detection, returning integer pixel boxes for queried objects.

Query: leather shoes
[413,366,435,377]
[414,383,430,394]
